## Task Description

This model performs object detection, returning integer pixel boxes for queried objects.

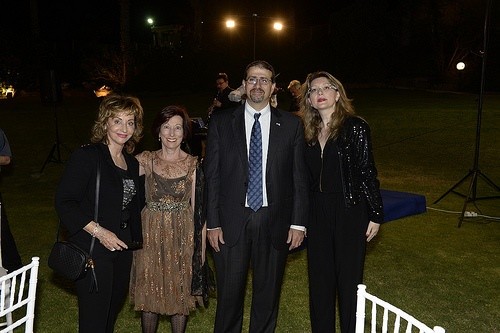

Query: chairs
[355,284,445,333]
[0,256,40,333]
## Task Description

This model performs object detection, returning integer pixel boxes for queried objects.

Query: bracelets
[93,223,99,237]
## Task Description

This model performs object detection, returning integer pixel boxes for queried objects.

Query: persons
[298,71,387,333]
[0,115,40,309]
[134,105,207,332]
[53,93,148,333]
[202,60,310,333]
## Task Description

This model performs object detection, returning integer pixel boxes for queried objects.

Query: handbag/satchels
[47,239,99,298]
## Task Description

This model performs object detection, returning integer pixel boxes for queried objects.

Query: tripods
[434,0,500,228]
[39,71,72,172]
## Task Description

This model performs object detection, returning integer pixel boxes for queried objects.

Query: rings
[111,248,116,252]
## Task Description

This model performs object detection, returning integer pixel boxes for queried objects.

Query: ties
[247,113,263,213]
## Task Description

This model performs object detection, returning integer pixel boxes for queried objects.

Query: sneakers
[216,82,225,86]
[245,77,272,84]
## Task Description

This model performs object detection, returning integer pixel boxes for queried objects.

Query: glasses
[307,83,337,94]
